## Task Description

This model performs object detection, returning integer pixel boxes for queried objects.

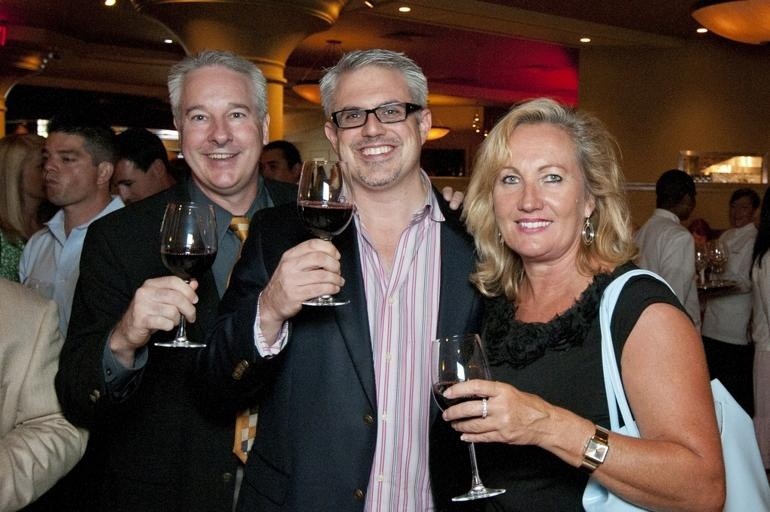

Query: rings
[482,399,487,419]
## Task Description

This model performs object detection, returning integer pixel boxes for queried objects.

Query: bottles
[692,175,713,183]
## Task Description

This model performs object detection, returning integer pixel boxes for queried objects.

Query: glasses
[326,100,424,129]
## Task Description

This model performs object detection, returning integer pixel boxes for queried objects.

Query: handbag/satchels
[575,265,770,512]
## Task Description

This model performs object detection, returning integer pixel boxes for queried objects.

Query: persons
[200,47,484,509]
[259,140,304,184]
[685,186,770,470]
[630,169,700,332]
[441,96,726,509]
[1,120,192,510]
[54,49,468,510]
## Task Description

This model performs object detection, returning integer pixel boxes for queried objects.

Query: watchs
[577,423,611,474]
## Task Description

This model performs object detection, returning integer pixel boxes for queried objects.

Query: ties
[226,216,248,284]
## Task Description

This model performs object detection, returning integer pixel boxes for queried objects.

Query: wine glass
[152,201,217,354]
[289,158,356,308]
[428,334,509,501]
[696,239,729,288]
